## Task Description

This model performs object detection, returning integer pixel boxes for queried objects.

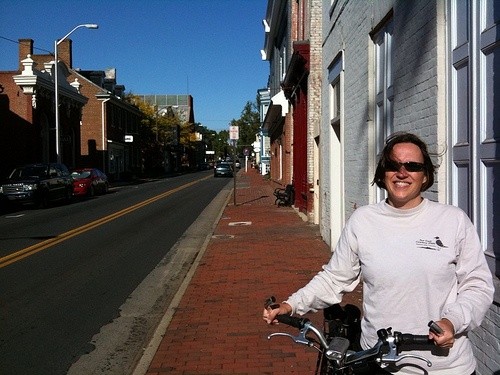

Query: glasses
[386,161,427,172]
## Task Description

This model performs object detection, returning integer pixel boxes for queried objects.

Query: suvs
[0,162,75,210]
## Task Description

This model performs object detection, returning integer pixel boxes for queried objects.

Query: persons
[264,132,495,375]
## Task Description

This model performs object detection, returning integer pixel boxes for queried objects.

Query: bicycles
[264,296,445,375]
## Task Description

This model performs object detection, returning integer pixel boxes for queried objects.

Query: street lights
[54,23,99,163]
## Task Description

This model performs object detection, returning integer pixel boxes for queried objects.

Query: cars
[214,164,233,177]
[200,161,216,169]
[71,169,109,196]
[236,159,241,169]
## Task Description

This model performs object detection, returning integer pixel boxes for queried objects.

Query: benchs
[273,184,295,208]
